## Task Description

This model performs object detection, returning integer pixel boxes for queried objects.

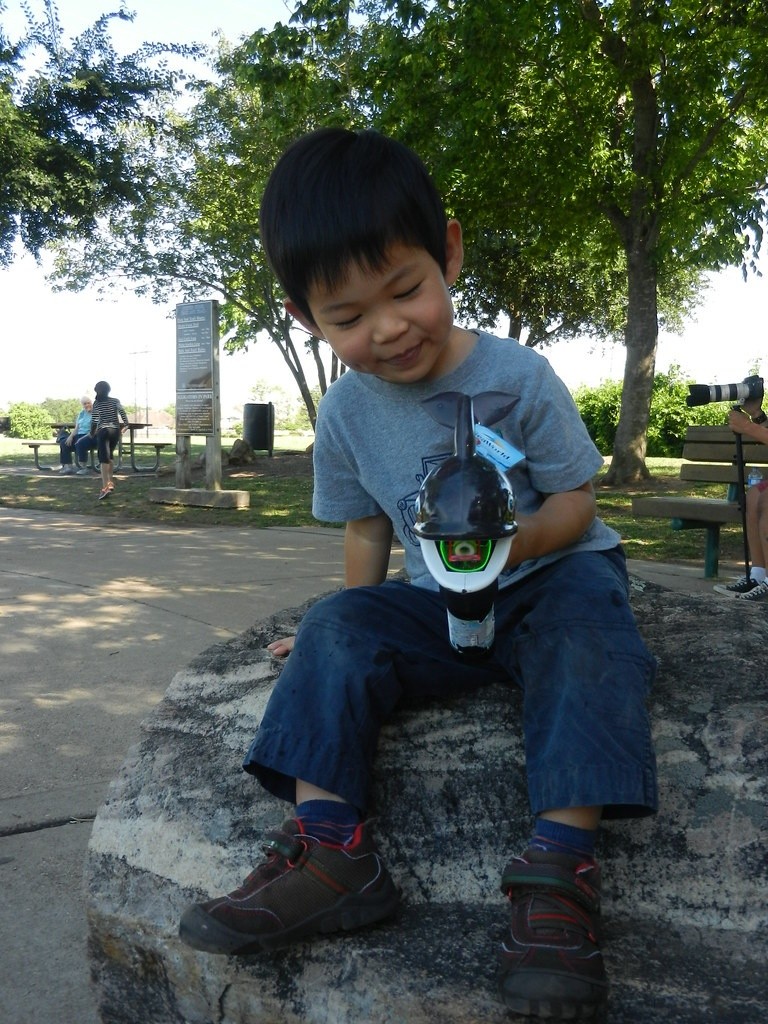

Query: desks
[51,422,151,475]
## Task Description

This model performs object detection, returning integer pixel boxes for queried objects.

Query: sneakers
[98,487,111,500]
[179,819,399,954]
[108,481,114,489]
[712,576,759,598]
[501,845,611,1019]
[729,580,768,602]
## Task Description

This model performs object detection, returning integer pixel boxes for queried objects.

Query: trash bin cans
[242,402,275,458]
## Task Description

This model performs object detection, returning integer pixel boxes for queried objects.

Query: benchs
[120,442,172,472]
[21,443,114,473]
[632,425,768,578]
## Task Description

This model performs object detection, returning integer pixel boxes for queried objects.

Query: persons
[89,381,130,501]
[711,377,768,602]
[59,397,97,477]
[178,131,659,1024]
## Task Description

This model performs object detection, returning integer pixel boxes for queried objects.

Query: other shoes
[59,468,73,473]
[76,469,89,474]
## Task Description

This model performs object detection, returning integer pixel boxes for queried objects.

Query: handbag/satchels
[56,425,71,443]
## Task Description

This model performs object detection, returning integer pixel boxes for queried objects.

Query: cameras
[686,375,764,407]
